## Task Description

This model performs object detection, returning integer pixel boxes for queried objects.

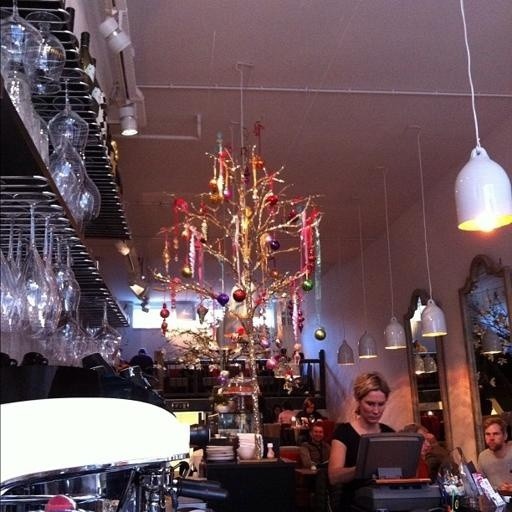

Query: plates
[205,445,233,461]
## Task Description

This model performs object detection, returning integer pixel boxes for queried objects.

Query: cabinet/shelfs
[0,0,131,326]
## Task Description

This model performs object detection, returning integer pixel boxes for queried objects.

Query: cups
[0,352,159,389]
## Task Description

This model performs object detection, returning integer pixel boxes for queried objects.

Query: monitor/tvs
[354,432,425,480]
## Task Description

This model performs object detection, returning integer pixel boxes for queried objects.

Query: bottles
[267,442,274,459]
[64,6,122,196]
[168,363,264,393]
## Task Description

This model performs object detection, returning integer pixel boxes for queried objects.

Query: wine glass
[1,0,102,244]
[1,193,123,367]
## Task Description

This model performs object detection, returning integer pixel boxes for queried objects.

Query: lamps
[454,0,512,234]
[378,162,407,349]
[98,17,131,56]
[336,240,353,365]
[216,61,262,350]
[274,240,301,379]
[355,196,378,359]
[413,297,437,375]
[479,272,501,355]
[119,103,138,136]
[410,126,447,338]
[115,239,129,257]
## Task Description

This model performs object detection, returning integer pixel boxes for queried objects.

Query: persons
[279,401,298,424]
[398,423,429,483]
[301,422,331,471]
[328,373,395,506]
[295,397,322,425]
[271,404,282,424]
[478,418,512,493]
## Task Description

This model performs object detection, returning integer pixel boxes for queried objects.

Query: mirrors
[403,289,452,451]
[458,254,512,459]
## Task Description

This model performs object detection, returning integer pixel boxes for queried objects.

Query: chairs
[420,416,440,442]
[178,419,344,512]
